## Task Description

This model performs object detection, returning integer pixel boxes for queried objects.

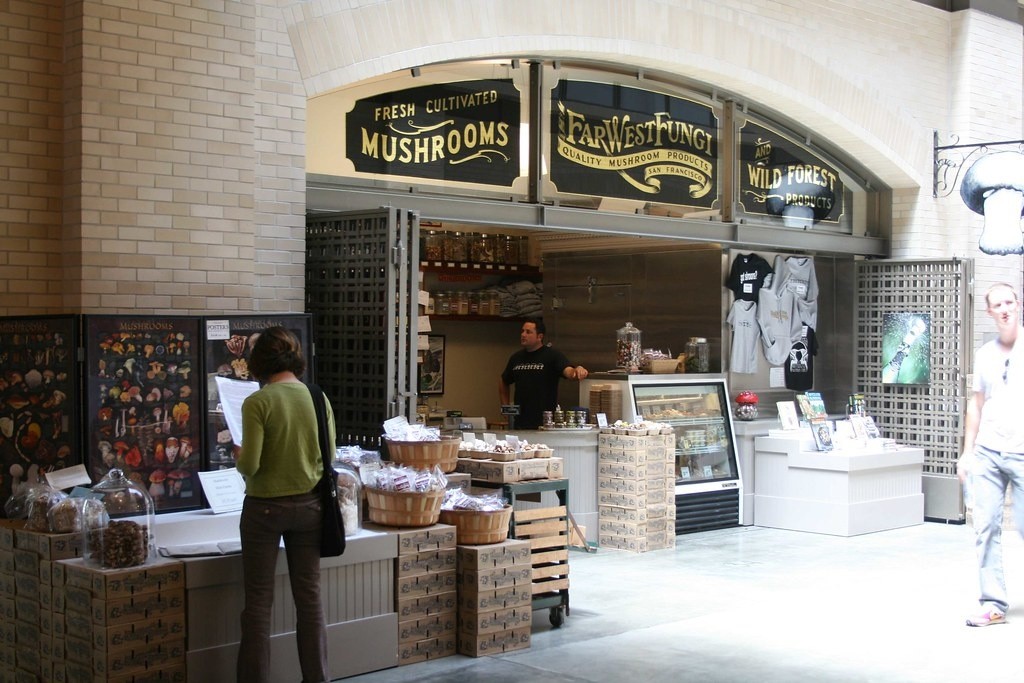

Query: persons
[954,281,1024,628]
[498,316,588,430]
[231,325,339,683]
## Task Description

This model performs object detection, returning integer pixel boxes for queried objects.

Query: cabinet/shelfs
[420,258,543,321]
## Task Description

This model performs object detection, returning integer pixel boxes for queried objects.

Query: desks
[113,508,398,683]
[754,436,924,537]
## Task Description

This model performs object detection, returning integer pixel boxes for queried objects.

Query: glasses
[1003,358,1009,380]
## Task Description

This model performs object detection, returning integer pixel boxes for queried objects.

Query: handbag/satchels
[319,467,346,558]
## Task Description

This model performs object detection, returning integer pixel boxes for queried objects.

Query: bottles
[677,336,710,373]
[847,393,867,418]
[882,342,910,383]
[81,467,156,569]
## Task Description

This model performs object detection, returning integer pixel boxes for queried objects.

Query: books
[836,413,898,453]
[775,400,800,430]
[796,391,829,422]
[810,422,834,451]
[848,393,867,418]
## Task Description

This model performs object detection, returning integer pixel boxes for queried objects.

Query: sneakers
[966,605,1006,627]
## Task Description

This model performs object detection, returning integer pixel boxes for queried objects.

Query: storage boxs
[361,523,531,667]
[458,457,564,483]
[598,426,677,552]
[0,518,186,683]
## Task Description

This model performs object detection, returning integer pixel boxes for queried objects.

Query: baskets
[600,427,673,436]
[459,449,555,461]
